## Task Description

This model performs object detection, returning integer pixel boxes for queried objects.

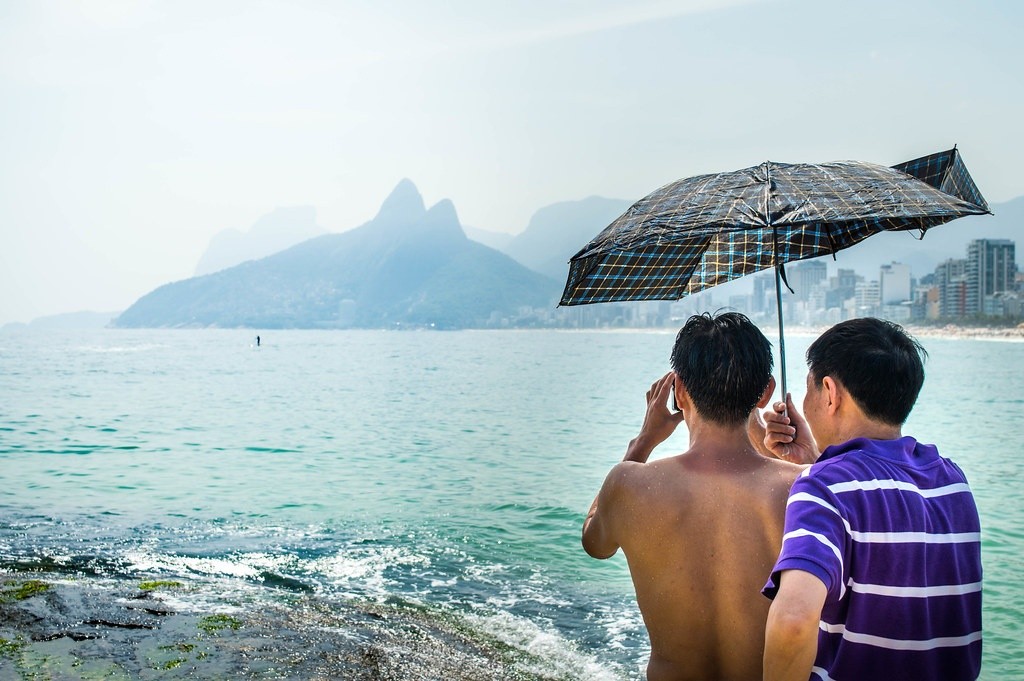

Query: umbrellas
[556,144,994,456]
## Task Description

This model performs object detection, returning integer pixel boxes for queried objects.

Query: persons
[761,318,982,681]
[581,312,814,681]
